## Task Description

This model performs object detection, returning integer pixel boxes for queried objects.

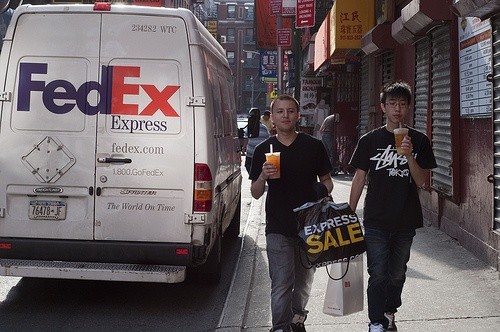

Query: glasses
[383,100,410,109]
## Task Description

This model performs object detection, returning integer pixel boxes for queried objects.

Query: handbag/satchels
[291,199,370,270]
[323,251,365,316]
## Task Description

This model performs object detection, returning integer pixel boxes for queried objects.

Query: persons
[249,94,334,332]
[348,82,438,332]
[260,111,273,134]
[312,97,339,176]
[245,108,269,175]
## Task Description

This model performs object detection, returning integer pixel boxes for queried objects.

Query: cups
[393,128,409,155]
[264,151,282,179]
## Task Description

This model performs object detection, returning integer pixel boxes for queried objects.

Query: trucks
[0,1,261,285]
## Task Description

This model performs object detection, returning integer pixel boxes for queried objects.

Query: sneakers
[368,320,388,332]
[383,312,397,332]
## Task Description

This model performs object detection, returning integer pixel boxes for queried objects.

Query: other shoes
[290,312,307,332]
